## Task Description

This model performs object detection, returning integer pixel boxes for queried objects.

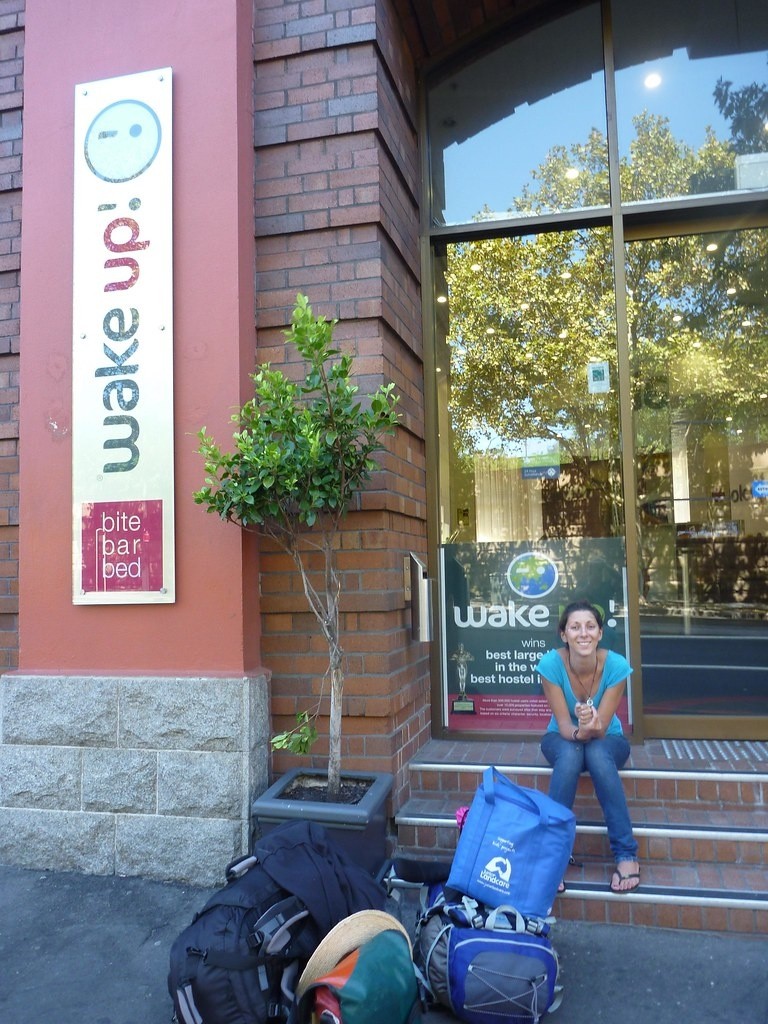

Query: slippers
[560,882,569,896]
[609,865,644,893]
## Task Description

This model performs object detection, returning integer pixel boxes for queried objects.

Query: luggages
[161,852,402,1021]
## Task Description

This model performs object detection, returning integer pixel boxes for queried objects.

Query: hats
[294,908,413,1006]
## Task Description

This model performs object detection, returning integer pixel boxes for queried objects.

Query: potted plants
[183,292,404,881]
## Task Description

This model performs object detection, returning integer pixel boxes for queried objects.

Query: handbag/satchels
[293,930,420,1024]
[410,877,567,1023]
[443,762,576,923]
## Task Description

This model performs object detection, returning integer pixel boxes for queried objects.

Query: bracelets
[571,727,581,741]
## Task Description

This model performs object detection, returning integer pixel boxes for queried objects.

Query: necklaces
[569,652,599,707]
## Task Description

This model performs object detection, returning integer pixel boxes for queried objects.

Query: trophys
[450,643,477,715]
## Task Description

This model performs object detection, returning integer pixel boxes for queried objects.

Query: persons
[535,600,642,893]
